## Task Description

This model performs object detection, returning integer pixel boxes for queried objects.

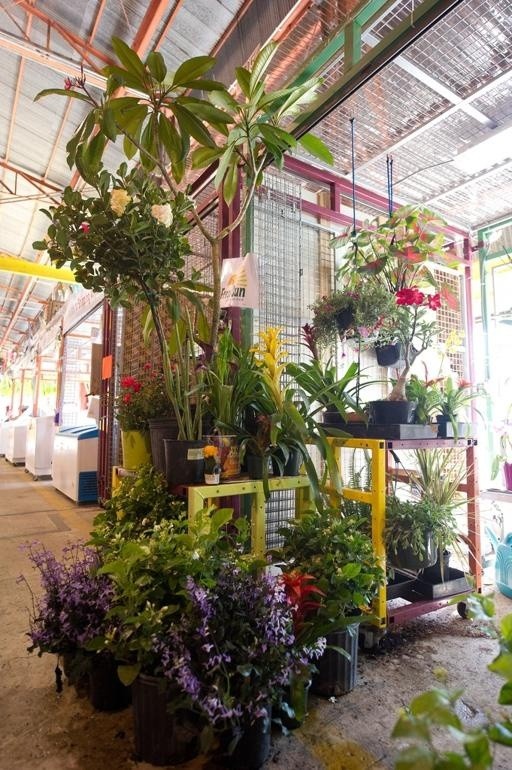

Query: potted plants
[369,459,484,603]
[27,37,337,492]
[19,504,268,710]
[306,197,474,446]
[271,506,393,703]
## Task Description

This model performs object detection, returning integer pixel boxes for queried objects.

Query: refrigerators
[50,424,100,504]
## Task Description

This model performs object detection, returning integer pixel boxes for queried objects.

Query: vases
[122,673,277,768]
